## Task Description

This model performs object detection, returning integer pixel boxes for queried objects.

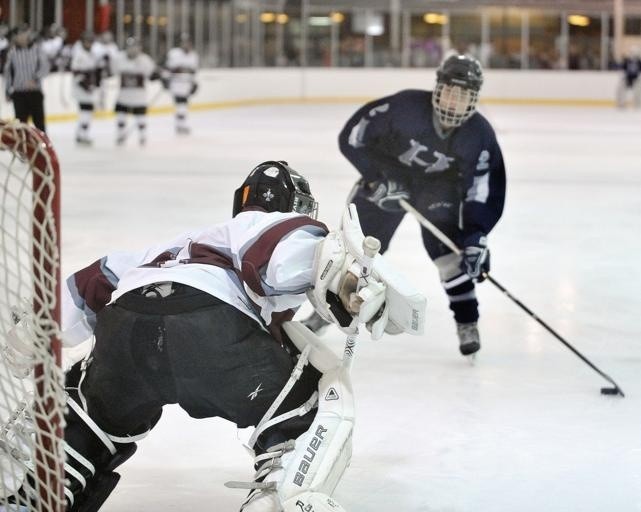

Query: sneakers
[453,315,483,357]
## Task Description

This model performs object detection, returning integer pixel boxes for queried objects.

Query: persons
[3,21,47,132]
[411,40,599,71]
[2,159,433,511]
[159,28,201,134]
[337,51,507,356]
[614,44,641,110]
[107,36,170,154]
[39,26,122,72]
[69,31,113,147]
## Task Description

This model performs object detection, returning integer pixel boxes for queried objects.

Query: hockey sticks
[400,198,626,399]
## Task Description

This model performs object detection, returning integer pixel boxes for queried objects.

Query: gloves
[459,228,493,286]
[189,83,199,96]
[361,177,414,214]
[310,248,390,344]
[161,76,171,89]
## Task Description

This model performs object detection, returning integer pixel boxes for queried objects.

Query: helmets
[231,158,315,221]
[435,53,485,93]
